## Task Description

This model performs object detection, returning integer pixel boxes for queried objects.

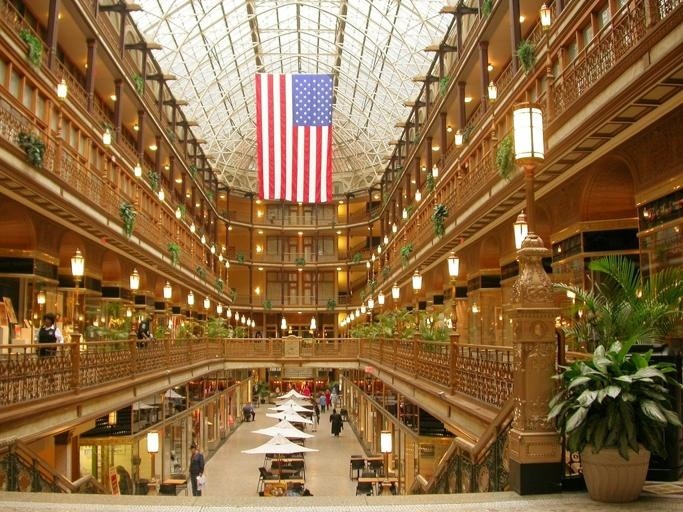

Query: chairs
[349,454,397,496]
[259,419,309,496]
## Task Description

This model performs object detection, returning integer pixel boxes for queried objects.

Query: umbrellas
[241,388,320,481]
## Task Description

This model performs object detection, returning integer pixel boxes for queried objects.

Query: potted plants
[545,253,683,503]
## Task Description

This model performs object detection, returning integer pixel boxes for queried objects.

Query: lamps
[146,431,159,453]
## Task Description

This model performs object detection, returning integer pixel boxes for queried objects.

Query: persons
[312,388,343,437]
[256,73,335,205]
[35,313,64,355]
[185,446,205,496]
[136,313,155,347]
[115,465,133,495]
